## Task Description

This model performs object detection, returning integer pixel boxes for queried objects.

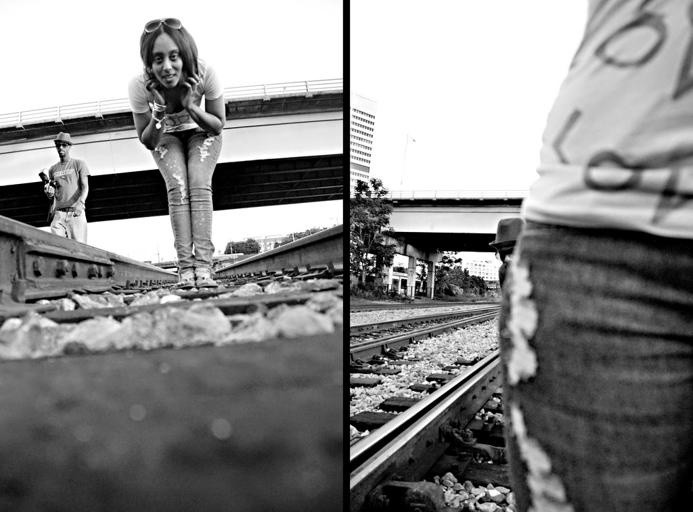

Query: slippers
[172,277,196,289]
[196,276,219,288]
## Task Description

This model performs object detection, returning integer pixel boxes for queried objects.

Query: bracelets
[78,199,85,204]
[151,102,167,129]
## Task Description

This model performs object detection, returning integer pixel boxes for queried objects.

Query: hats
[54,131,73,144]
[489,218,523,249]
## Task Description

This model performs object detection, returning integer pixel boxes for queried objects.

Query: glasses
[56,144,68,147]
[498,248,512,261]
[143,17,183,35]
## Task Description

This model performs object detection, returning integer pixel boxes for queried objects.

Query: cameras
[38,168,61,190]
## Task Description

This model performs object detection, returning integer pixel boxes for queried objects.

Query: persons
[43,132,90,244]
[127,17,226,291]
[492,0,693,512]
[488,218,527,295]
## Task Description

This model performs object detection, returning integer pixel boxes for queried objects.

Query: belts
[60,207,76,212]
